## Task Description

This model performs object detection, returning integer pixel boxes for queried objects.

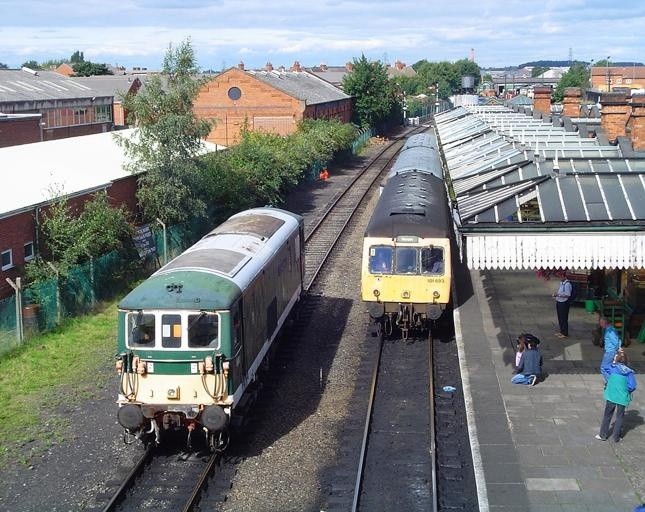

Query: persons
[511,340,545,386]
[515,343,527,367]
[551,271,573,338]
[595,350,637,443]
[598,317,625,390]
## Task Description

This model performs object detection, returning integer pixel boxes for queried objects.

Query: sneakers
[554,331,568,339]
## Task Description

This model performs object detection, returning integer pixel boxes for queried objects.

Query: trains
[361,134,451,343]
[114,207,305,453]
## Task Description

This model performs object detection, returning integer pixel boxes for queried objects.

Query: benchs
[595,286,633,325]
